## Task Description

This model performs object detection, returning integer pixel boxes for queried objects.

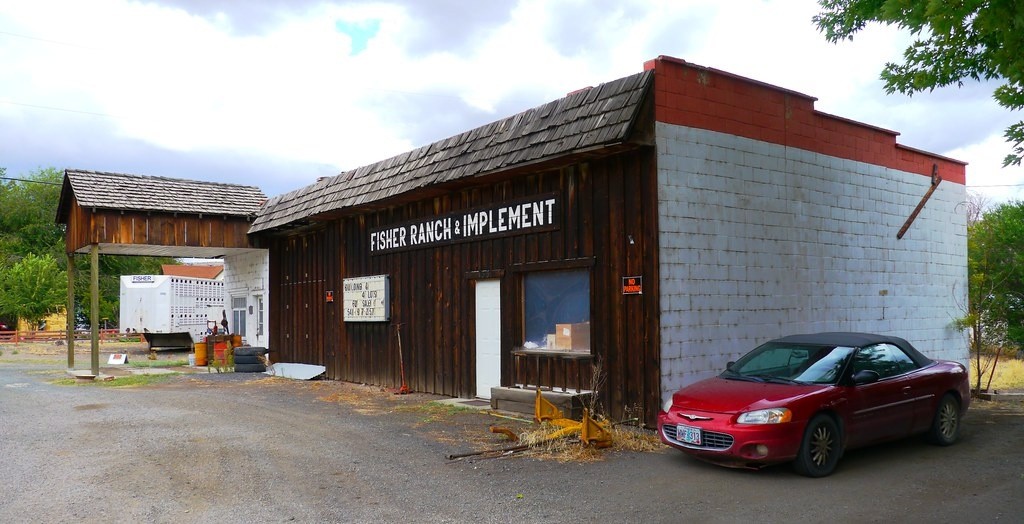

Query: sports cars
[656,330,972,479]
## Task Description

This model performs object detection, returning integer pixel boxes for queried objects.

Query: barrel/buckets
[188,334,242,367]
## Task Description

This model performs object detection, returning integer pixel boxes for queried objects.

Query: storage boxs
[555,322,589,350]
